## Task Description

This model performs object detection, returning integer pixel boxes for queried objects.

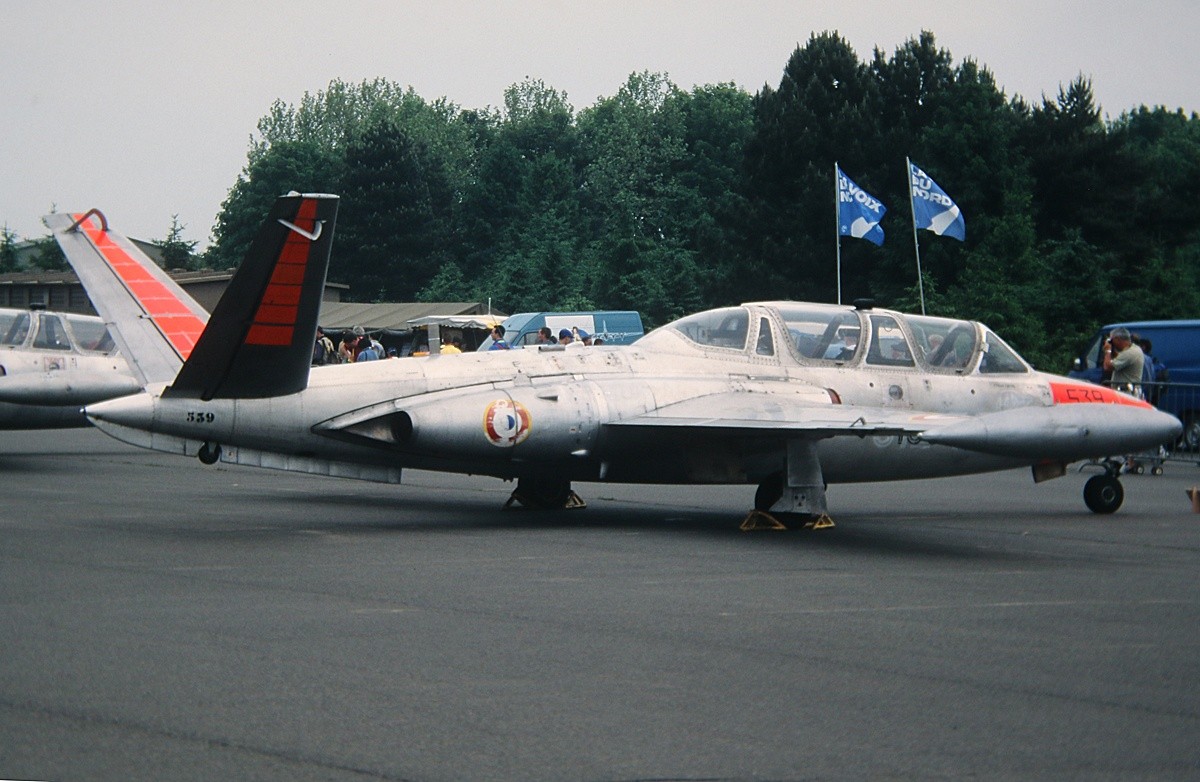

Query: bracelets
[1105,349,1112,353]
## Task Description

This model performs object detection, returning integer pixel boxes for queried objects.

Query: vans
[479,310,644,353]
[1065,319,1200,455]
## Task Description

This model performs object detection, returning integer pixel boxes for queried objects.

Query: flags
[910,163,965,241]
[839,169,886,246]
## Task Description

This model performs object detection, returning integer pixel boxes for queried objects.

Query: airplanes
[0,304,144,406]
[38,191,1185,536]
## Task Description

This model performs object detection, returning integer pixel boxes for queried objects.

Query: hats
[559,329,575,338]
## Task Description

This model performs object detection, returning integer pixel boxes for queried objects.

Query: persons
[538,327,558,344]
[440,333,462,354]
[356,338,378,362]
[824,329,849,358]
[845,336,858,349]
[582,337,592,346]
[344,331,363,362]
[1130,333,1155,401]
[556,329,574,345]
[594,339,605,345]
[369,332,385,358]
[338,330,353,353]
[1140,339,1170,465]
[488,325,510,350]
[1104,328,1146,473]
[312,326,334,365]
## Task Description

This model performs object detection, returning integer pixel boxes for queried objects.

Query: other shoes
[1120,463,1137,473]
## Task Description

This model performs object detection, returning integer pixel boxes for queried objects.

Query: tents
[318,301,510,351]
[407,315,510,330]
